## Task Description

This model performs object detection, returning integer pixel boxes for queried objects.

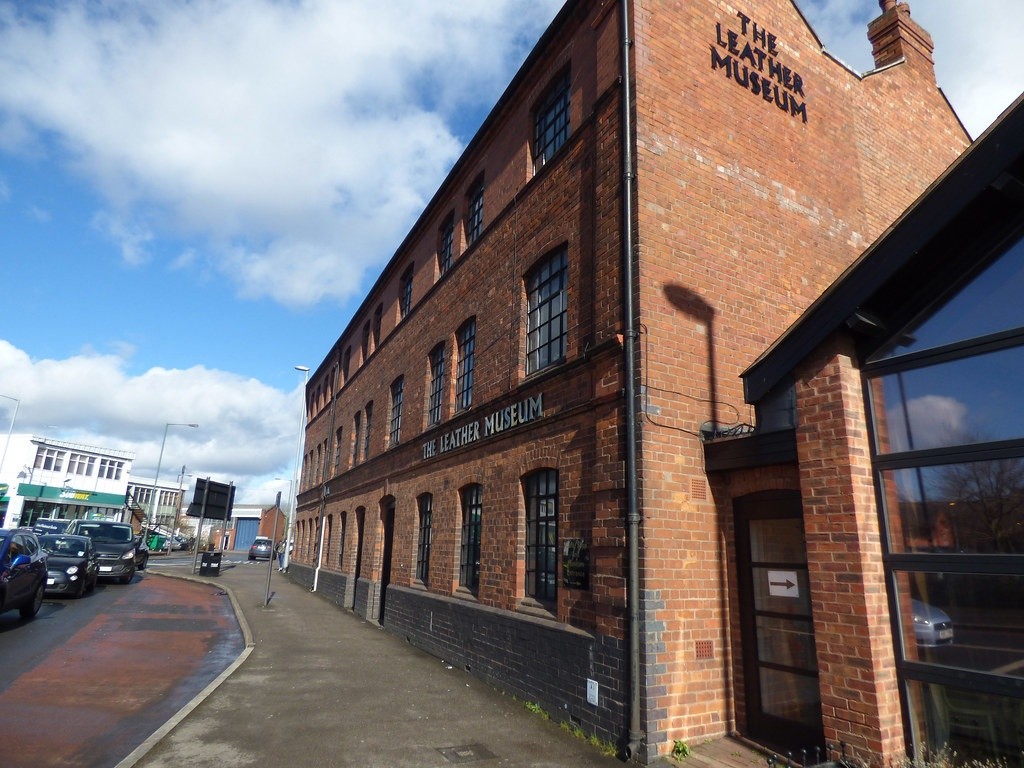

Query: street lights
[142,423,199,545]
[283,366,319,573]
[275,477,292,540]
[167,474,192,555]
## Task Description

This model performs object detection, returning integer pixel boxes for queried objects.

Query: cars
[278,540,294,555]
[32,517,71,536]
[74,520,137,585]
[0,528,48,618]
[248,538,277,561]
[911,599,954,647]
[135,534,149,570]
[37,534,99,600]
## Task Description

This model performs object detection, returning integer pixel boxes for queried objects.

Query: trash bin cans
[209,543,214,551]
[149,534,167,551]
[199,551,222,578]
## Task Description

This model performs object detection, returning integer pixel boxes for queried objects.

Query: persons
[189,535,195,554]
[274,537,287,571]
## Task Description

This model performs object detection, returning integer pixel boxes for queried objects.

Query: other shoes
[278,568,283,571]
